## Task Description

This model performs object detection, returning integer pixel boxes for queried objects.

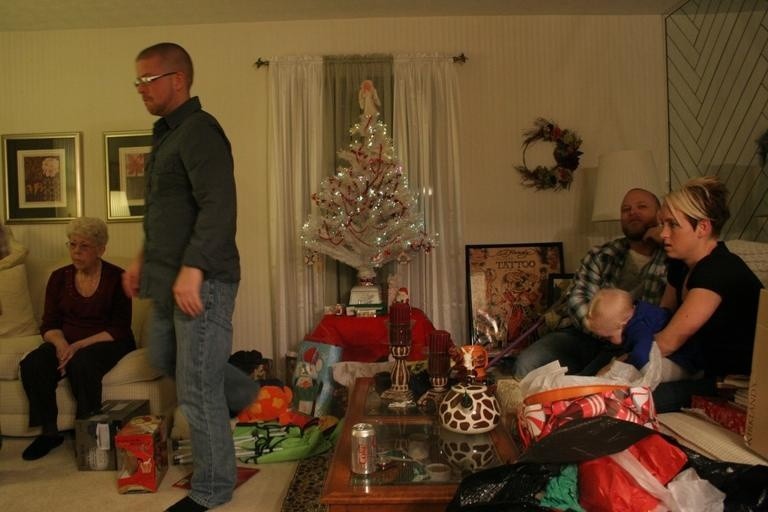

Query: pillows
[0,262,42,341]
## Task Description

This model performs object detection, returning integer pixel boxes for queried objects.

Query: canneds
[351,423,377,478]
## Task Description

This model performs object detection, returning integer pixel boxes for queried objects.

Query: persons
[596,174,764,415]
[124,43,260,511]
[584,287,699,386]
[19,215,137,461]
[513,188,673,379]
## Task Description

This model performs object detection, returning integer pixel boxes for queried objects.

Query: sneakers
[20,428,64,461]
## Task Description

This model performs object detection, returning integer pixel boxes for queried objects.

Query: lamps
[587,149,664,225]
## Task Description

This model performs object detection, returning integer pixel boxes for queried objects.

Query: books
[717,374,749,411]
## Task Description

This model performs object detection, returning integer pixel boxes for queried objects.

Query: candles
[425,328,451,373]
[387,299,413,345]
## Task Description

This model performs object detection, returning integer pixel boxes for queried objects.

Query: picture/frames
[464,239,567,363]
[102,130,156,225]
[547,272,574,299]
[1,130,86,226]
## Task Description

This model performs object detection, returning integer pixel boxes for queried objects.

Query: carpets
[274,386,348,511]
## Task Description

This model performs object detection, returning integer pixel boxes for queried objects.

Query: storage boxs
[739,283,768,464]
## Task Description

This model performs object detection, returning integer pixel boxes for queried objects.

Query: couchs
[497,236,768,463]
[0,252,179,441]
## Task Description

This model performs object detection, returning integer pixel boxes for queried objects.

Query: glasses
[64,238,99,251]
[132,70,175,88]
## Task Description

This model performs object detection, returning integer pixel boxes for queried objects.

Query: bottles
[436,374,502,435]
[437,431,496,478]
